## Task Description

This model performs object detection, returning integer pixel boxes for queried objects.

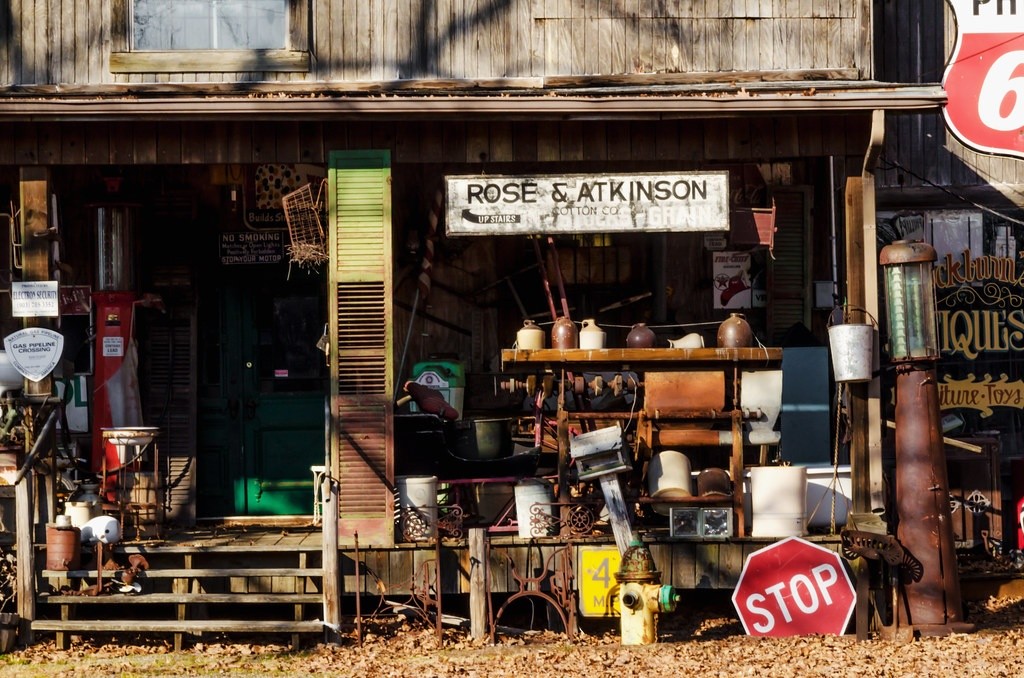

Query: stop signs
[731,536,857,638]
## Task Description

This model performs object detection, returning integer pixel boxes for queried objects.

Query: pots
[443,417,515,459]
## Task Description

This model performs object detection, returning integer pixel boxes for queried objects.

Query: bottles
[516,320,545,349]
[668,333,705,349]
[552,316,579,349]
[717,313,753,348]
[626,324,658,348]
[579,319,607,349]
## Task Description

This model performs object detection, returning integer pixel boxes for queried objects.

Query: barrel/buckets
[751,466,809,538]
[513,478,552,539]
[649,449,693,516]
[825,303,879,382]
[395,474,437,542]
[695,467,732,497]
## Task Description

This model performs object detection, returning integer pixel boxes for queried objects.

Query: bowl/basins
[101,427,159,445]
